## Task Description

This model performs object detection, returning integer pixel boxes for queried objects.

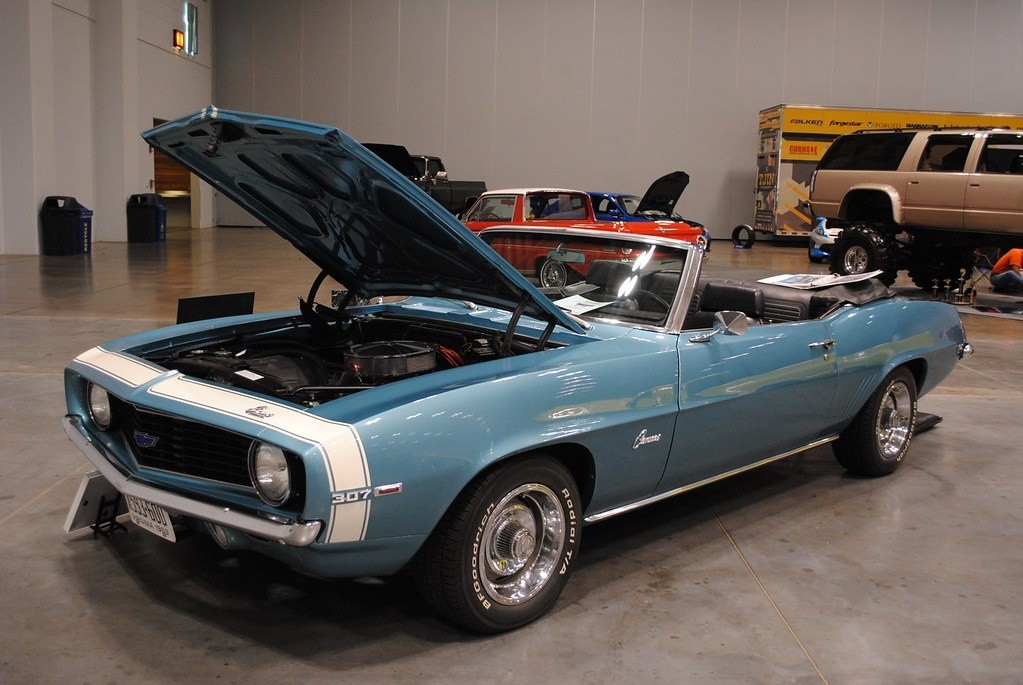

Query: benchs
[642,272,817,325]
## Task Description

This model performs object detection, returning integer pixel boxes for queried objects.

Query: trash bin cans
[38,194,94,254]
[126,192,167,241]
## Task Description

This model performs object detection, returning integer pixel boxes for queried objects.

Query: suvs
[807,125,1023,290]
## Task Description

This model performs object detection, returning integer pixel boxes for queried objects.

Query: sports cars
[456,171,711,289]
[63,102,975,636]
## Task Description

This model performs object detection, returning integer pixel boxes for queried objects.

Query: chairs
[691,282,765,330]
[586,260,641,313]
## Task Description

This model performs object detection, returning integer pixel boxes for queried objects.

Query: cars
[809,216,844,265]
